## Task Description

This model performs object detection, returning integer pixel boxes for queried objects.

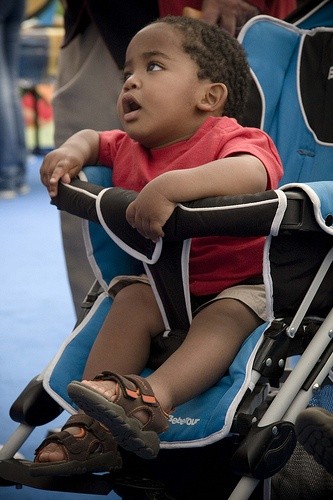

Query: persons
[24,14,286,478]
[0,1,261,330]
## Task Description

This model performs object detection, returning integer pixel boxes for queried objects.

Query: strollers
[0,0,333,500]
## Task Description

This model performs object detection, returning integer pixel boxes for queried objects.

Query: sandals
[27,414,121,476]
[67,370,169,459]
